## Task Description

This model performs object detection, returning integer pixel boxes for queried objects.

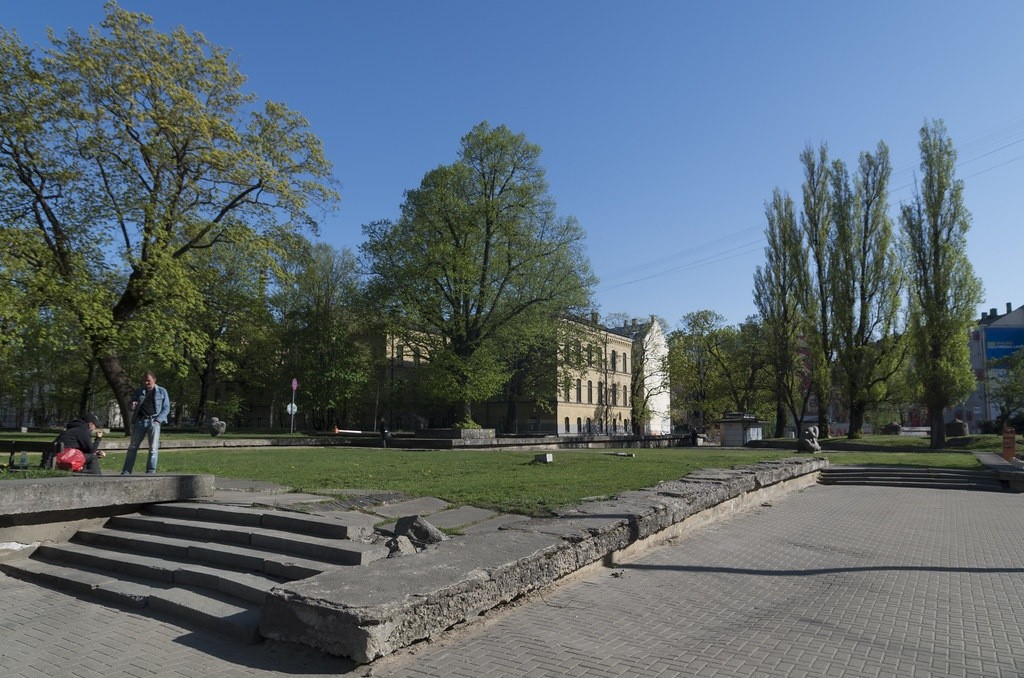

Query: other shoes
[121,470,129,475]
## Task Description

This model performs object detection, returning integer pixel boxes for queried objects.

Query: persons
[121,371,170,474]
[690,427,700,447]
[54,414,106,474]
[379,418,392,448]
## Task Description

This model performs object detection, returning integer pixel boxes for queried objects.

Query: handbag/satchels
[57,449,86,472]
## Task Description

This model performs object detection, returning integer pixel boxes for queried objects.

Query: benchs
[0,439,77,474]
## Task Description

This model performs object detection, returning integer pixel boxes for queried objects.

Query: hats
[83,414,102,426]
